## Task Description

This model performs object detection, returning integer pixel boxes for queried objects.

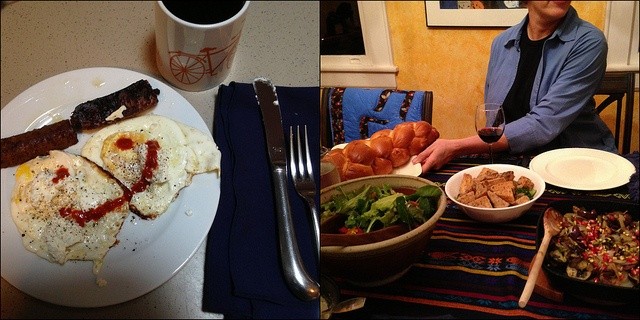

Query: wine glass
[476,102,506,169]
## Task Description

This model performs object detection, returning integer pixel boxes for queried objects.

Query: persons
[413,0,620,175]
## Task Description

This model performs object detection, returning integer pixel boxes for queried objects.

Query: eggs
[84,113,223,219]
[10,150,129,286]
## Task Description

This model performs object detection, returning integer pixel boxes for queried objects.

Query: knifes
[252,77,319,300]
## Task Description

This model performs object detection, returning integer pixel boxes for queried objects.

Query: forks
[288,123,321,263]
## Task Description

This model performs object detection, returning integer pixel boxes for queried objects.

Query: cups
[154,0,249,93]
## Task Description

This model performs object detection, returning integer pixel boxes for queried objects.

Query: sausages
[1,118,78,168]
[71,80,159,133]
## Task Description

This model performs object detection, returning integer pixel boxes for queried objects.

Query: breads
[319,120,439,182]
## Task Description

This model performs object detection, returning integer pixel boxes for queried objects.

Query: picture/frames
[425,0,528,27]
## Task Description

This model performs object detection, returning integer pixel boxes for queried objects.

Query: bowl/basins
[444,161,546,222]
[320,174,446,253]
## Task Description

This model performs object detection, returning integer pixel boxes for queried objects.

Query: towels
[202,81,320,320]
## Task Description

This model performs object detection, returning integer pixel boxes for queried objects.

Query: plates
[334,138,422,180]
[534,197,640,291]
[529,145,637,192]
[1,65,221,309]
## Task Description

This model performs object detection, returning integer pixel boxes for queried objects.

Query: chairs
[592,70,640,155]
[321,88,433,147]
